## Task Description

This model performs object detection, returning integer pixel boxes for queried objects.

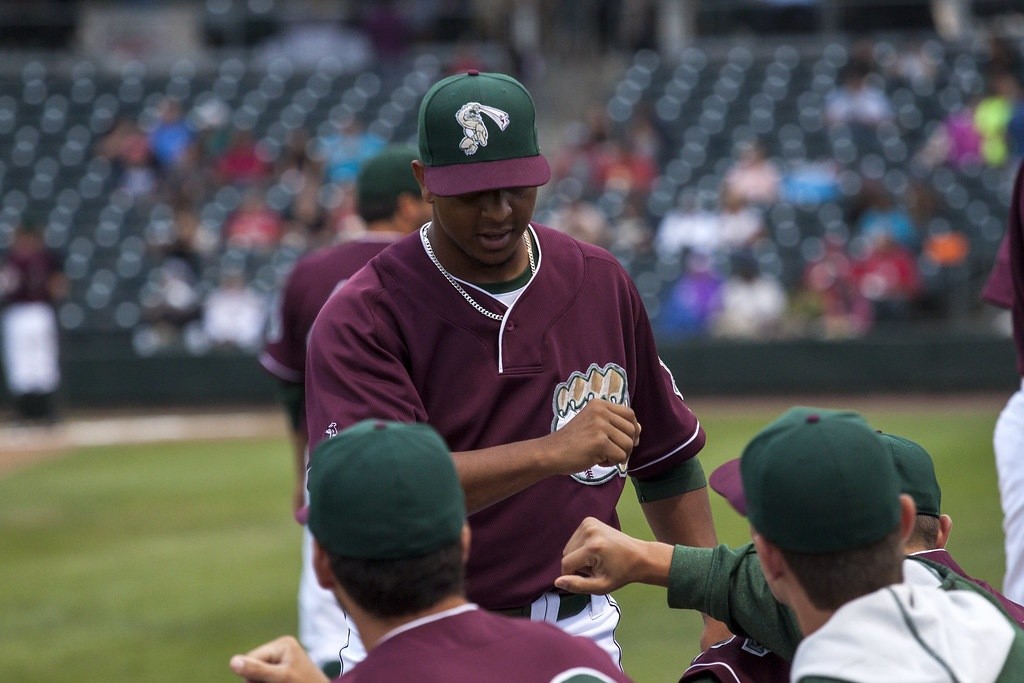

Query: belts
[496,585,590,622]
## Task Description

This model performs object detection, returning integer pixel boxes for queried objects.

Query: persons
[256,158,429,681]
[307,70,736,679]
[0,22,1023,426]
[231,422,630,681]
[551,388,1016,679]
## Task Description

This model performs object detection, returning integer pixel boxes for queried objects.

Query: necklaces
[422,224,535,320]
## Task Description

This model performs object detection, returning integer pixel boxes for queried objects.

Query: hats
[307,419,466,559]
[876,429,942,518]
[416,68,551,196]
[709,406,901,550]
